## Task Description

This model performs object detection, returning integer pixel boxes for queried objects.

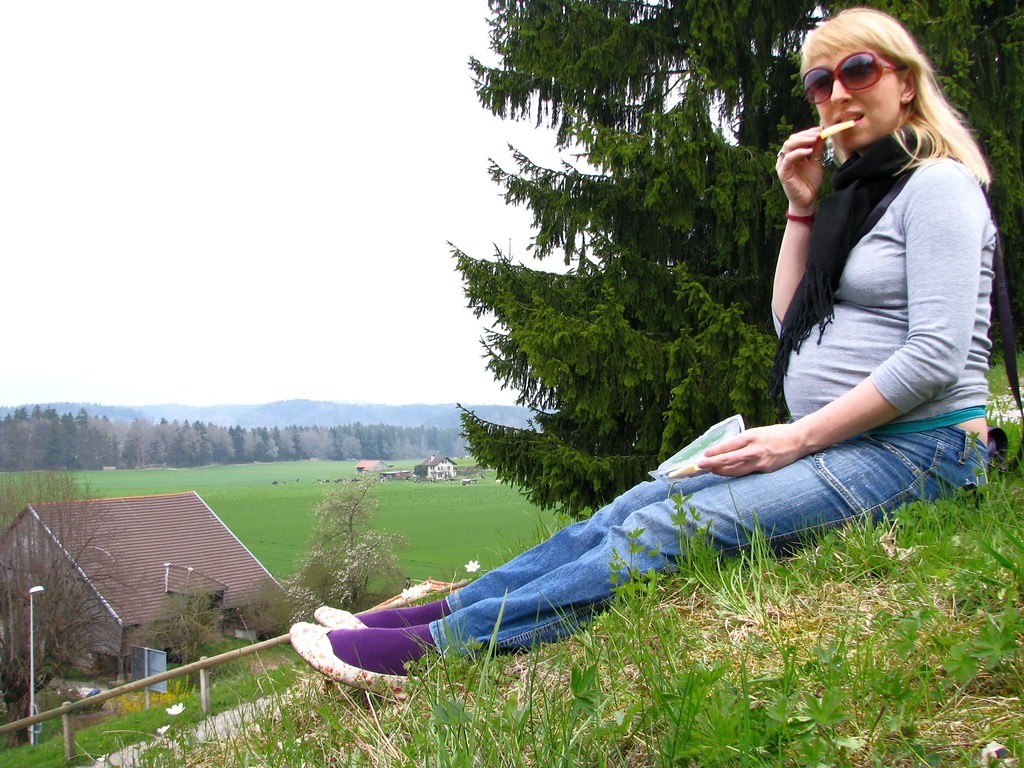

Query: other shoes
[314,605,368,630]
[289,620,424,700]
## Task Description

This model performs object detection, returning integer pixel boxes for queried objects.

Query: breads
[670,463,699,477]
[821,119,858,139]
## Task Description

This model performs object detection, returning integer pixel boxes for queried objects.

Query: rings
[810,154,825,163]
[776,149,788,158]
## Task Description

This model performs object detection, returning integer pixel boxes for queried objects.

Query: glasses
[801,53,908,104]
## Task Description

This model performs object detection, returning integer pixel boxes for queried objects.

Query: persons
[288,7,1000,704]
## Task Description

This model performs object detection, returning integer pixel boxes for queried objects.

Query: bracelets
[785,208,817,230]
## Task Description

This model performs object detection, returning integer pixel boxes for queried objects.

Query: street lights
[29,585,45,745]
